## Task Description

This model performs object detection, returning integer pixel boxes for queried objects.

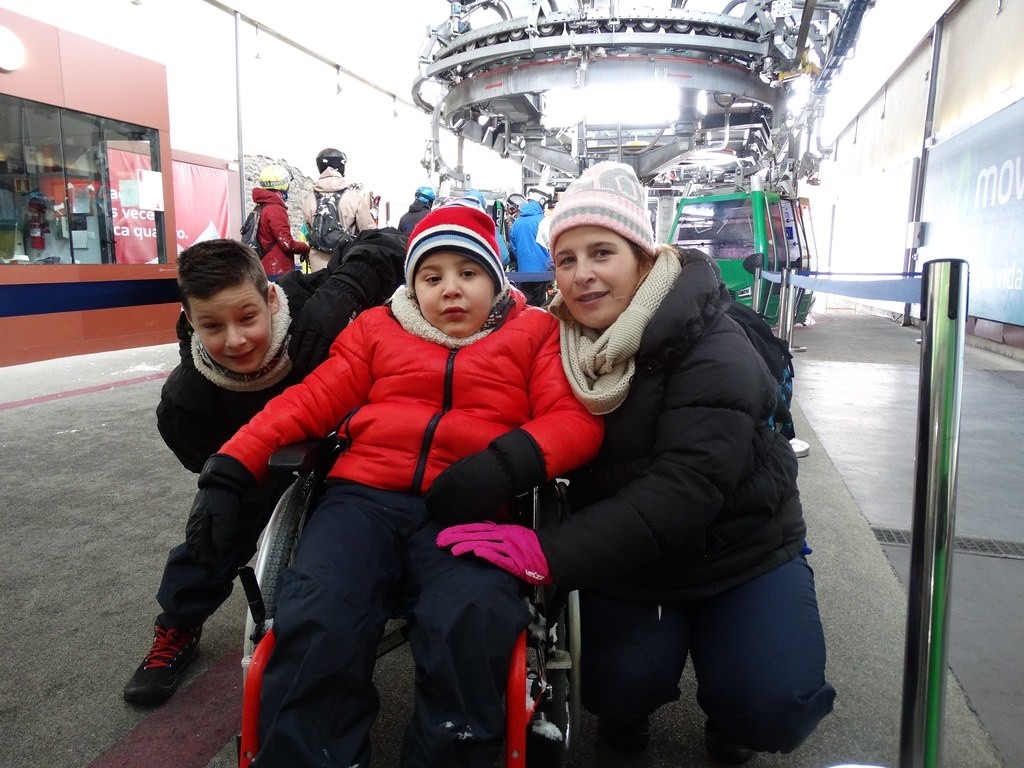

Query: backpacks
[716,282,797,441]
[239,201,279,263]
[307,189,357,255]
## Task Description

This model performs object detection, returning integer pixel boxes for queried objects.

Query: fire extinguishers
[21,187,45,250]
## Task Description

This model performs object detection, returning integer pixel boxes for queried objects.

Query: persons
[201,204,605,767]
[122,238,408,715]
[239,147,558,306]
[436,158,836,768]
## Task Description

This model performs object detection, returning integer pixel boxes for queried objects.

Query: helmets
[504,194,526,215]
[415,187,434,204]
[256,164,295,194]
[315,148,345,171]
[526,185,550,208]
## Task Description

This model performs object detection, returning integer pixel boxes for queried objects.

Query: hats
[549,159,656,262]
[403,190,509,300]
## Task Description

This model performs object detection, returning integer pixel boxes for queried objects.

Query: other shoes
[595,710,652,751]
[703,716,755,764]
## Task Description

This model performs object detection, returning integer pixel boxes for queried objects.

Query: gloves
[424,427,545,524]
[185,454,249,572]
[289,272,369,381]
[434,521,551,587]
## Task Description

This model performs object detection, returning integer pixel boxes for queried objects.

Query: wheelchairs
[235,435,581,768]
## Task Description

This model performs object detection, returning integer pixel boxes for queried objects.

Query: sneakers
[122,619,201,708]
